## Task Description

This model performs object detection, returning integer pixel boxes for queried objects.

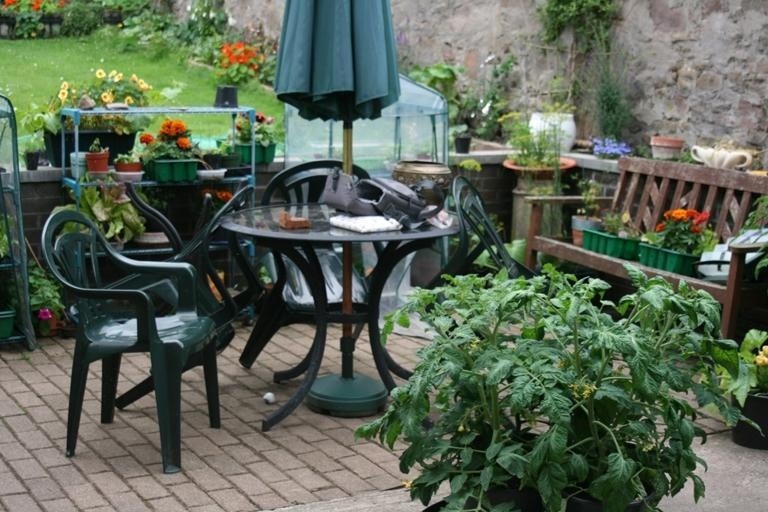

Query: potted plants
[526,65,582,156]
[84,136,111,173]
[509,276,763,509]
[390,263,567,511]
[579,207,640,261]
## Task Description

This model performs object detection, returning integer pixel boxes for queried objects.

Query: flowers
[643,196,713,255]
[138,115,202,160]
[571,175,605,220]
[46,67,153,135]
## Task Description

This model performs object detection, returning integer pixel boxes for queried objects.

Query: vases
[146,156,198,181]
[41,126,143,167]
[638,238,700,276]
[649,129,688,160]
[570,215,602,247]
[391,158,455,213]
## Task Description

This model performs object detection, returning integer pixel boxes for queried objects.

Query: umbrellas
[274,1,400,417]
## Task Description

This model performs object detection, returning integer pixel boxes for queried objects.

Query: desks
[218,203,461,434]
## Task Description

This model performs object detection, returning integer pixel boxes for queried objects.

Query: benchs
[518,146,767,360]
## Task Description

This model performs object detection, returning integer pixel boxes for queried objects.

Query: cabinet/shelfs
[1,92,38,355]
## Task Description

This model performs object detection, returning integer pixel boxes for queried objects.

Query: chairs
[237,165,372,374]
[41,206,223,477]
[422,175,544,307]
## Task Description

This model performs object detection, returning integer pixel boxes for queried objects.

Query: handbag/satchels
[319,167,425,219]
